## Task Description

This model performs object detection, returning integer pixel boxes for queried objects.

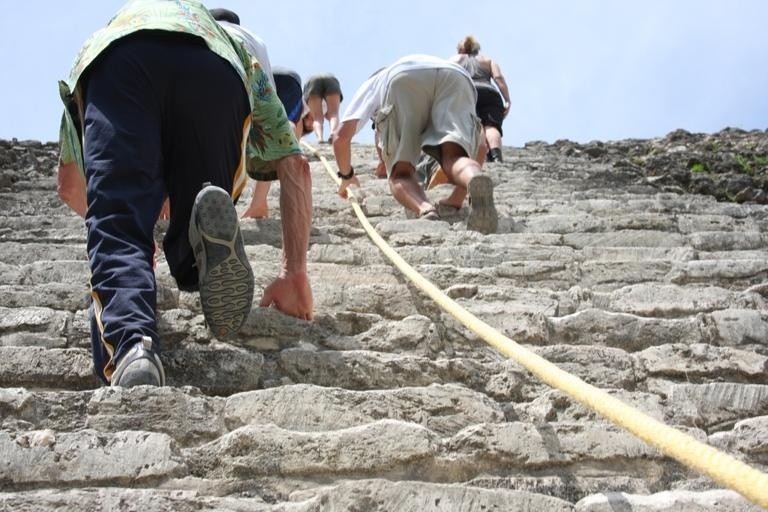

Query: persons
[450,34,511,163]
[302,72,344,144]
[333,54,500,236]
[55,1,315,390]
[208,7,276,219]
[272,69,310,147]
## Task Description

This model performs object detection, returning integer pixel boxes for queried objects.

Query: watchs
[337,166,355,180]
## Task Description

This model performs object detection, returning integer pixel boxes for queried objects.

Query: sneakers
[467,174,498,234]
[426,165,448,190]
[419,209,439,221]
[190,187,253,340]
[110,338,166,386]
